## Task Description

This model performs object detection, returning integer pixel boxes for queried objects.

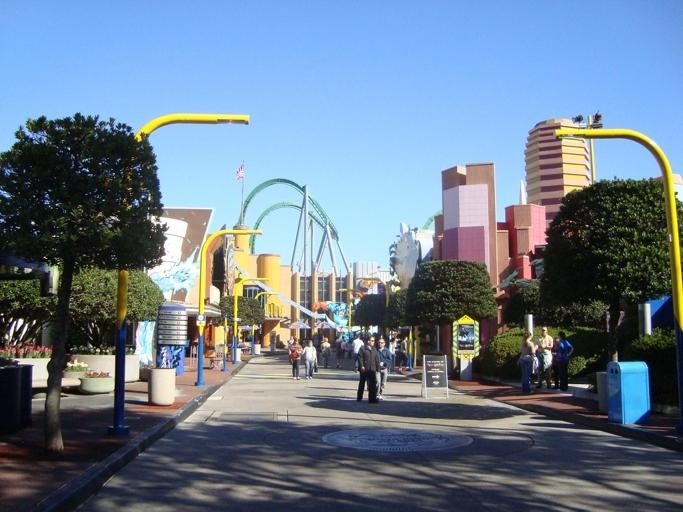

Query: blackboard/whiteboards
[423,354,448,389]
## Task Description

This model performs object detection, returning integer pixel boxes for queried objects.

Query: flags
[234,164,243,181]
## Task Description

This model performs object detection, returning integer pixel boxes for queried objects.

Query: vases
[62,369,84,380]
[79,373,115,395]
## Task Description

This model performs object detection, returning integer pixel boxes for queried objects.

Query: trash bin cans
[231,347,242,362]
[149,368,176,406]
[253,344,262,355]
[596,361,651,425]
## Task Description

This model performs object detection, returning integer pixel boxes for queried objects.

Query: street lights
[195,229,263,387]
[250,291,282,355]
[355,275,389,339]
[339,289,352,328]
[108,113,250,437]
[233,277,270,364]
[554,128,682,436]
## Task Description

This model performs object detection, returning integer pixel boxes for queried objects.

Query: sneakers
[528,385,568,394]
[357,395,384,403]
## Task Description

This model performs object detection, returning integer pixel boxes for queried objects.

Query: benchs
[10,358,81,396]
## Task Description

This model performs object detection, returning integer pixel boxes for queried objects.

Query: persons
[375,338,392,400]
[532,338,542,384]
[551,336,560,389]
[286,332,406,382]
[356,336,378,404]
[536,326,553,390]
[519,330,538,393]
[555,331,574,393]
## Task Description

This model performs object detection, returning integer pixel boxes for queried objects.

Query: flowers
[64,359,88,372]
[82,367,110,377]
